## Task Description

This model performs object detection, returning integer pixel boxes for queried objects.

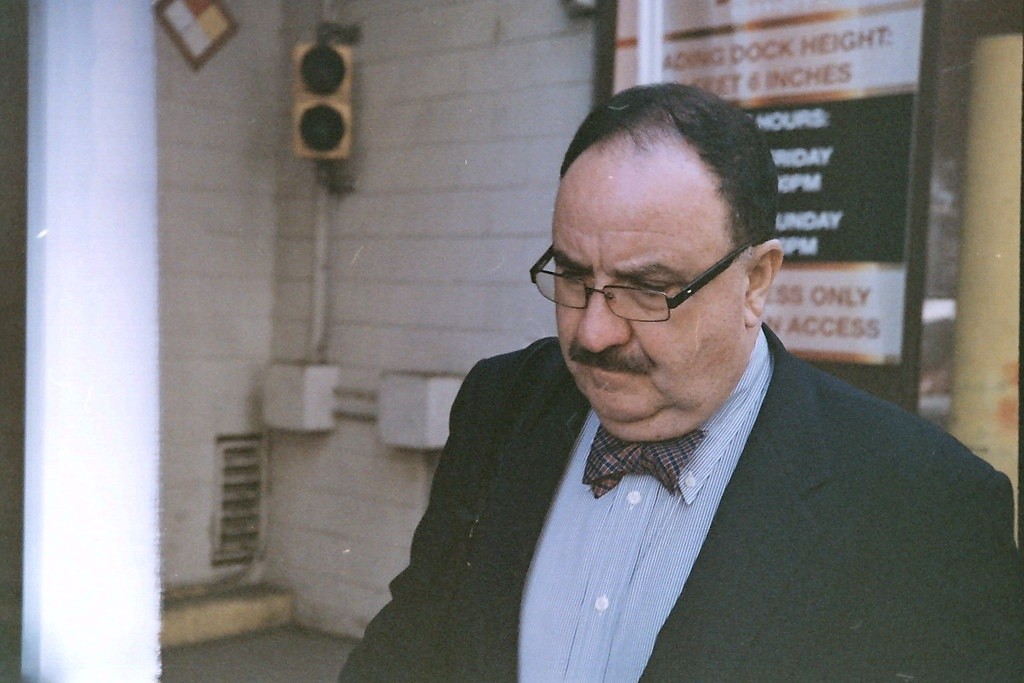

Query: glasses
[530,238,763,323]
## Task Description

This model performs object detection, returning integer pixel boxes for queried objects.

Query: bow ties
[582,423,710,500]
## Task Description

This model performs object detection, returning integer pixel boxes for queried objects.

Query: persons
[339,85,1023,683]
